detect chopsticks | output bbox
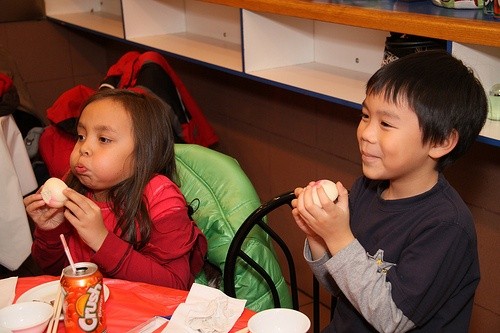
[46,284,62,333]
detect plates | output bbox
[15,280,110,320]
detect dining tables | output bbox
[0,274,258,332]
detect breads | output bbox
[41,177,69,208]
[310,179,338,208]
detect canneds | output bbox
[61,261,107,333]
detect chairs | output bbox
[222,187,350,331]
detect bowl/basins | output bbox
[247,308,311,333]
[0,301,54,333]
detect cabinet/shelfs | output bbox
[43,1,499,148]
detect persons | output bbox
[24,89,207,292]
[291,49,490,333]
